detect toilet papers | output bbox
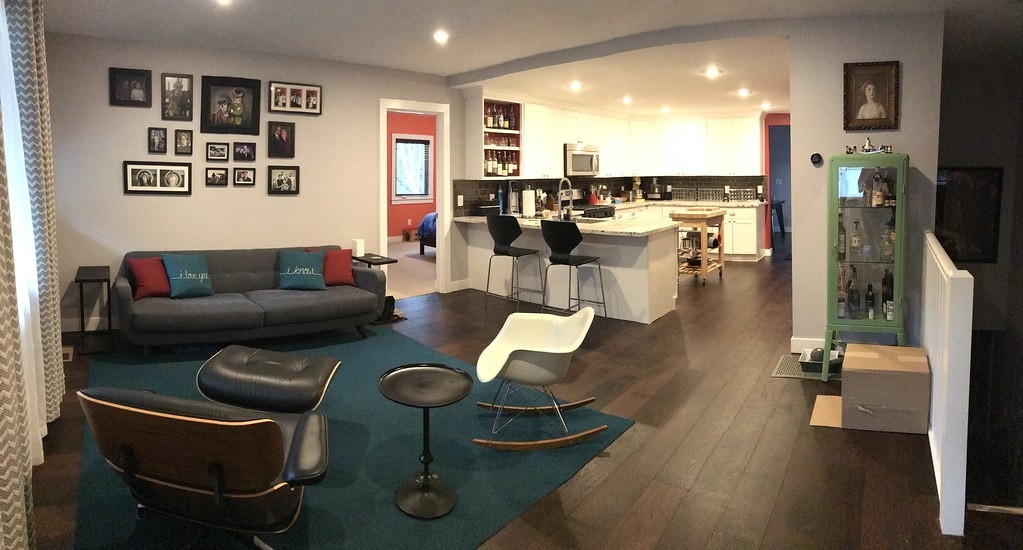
[522,189,536,217]
[352,239,365,257]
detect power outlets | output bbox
[407,219,413,226]
[621,185,625,192]
[489,194,495,201]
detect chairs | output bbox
[473,307,609,453]
[540,219,608,321]
[75,343,343,550]
[485,214,544,313]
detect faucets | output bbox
[557,177,573,221]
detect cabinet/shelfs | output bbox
[612,205,647,220]
[646,206,663,219]
[821,152,908,382]
[670,208,725,287]
[662,207,718,260]
[704,206,758,262]
[464,85,765,187]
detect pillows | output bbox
[324,250,355,286]
[161,252,215,298]
[278,249,327,292]
[126,257,170,300]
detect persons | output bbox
[115,78,146,102]
[273,171,296,192]
[177,133,191,152]
[211,173,216,183]
[237,171,251,182]
[275,91,316,109]
[165,81,189,116]
[151,130,163,151]
[141,172,150,185]
[237,145,252,160]
[271,126,291,156]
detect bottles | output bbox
[484,104,518,177]
[880,224,895,261]
[872,166,885,208]
[838,213,847,260]
[838,264,894,321]
[620,186,625,197]
[849,221,863,258]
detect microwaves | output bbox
[564,143,600,176]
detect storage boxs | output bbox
[401,228,420,242]
[645,192,673,200]
[798,347,840,373]
[808,342,930,435]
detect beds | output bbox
[417,212,437,256]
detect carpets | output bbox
[75,325,636,550]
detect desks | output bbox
[770,199,786,237]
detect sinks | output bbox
[572,217,607,224]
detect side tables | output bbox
[74,266,113,351]
[352,253,405,324]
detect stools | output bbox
[377,360,473,520]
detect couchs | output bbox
[114,244,387,359]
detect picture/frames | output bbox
[843,60,900,132]
[148,127,167,154]
[938,165,1005,264]
[205,167,228,187]
[174,128,193,156]
[233,167,256,186]
[206,141,230,162]
[122,160,194,195]
[268,80,323,116]
[233,141,258,161]
[267,165,300,194]
[200,75,262,136]
[267,120,296,160]
[108,67,153,109]
[161,72,194,122]
